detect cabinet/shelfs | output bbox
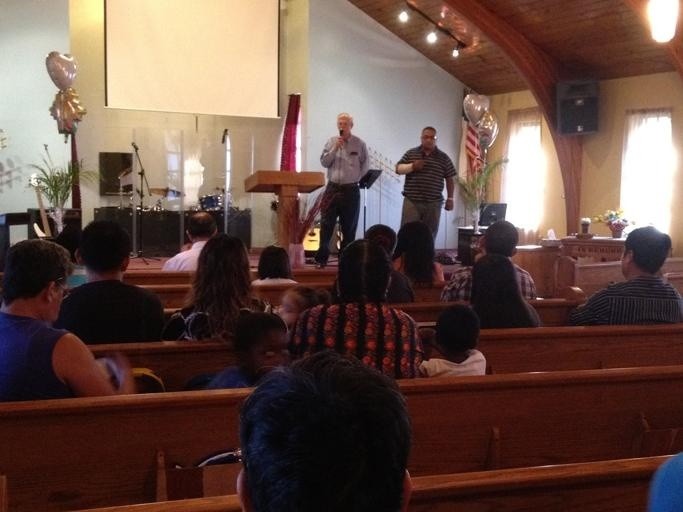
[27,206,83,240]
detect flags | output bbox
[456,111,488,207]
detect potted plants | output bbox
[276,185,340,271]
[450,157,509,234]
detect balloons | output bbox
[46,50,86,136]
[465,91,498,152]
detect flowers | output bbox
[590,207,637,226]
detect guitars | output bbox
[30,173,52,237]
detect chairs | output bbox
[0,268,683,511]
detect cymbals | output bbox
[150,187,185,198]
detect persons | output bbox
[464,253,546,327]
[393,223,446,283]
[164,235,273,344]
[238,351,414,512]
[570,227,683,325]
[395,126,456,235]
[316,115,370,270]
[295,240,423,377]
[442,222,538,301]
[0,239,135,401]
[54,220,167,343]
[367,224,397,256]
[274,285,330,332]
[252,245,301,284]
[421,306,486,377]
[204,313,290,388]
[164,214,218,270]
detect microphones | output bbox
[131,142,139,151]
[339,127,343,136]
[221,129,228,144]
[195,446,243,467]
[420,152,426,160]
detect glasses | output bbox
[420,135,437,140]
[54,280,70,299]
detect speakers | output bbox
[556,79,600,137]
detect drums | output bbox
[199,193,224,211]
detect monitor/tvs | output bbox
[478,203,507,226]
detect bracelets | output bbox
[446,197,454,200]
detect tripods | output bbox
[131,170,162,266]
